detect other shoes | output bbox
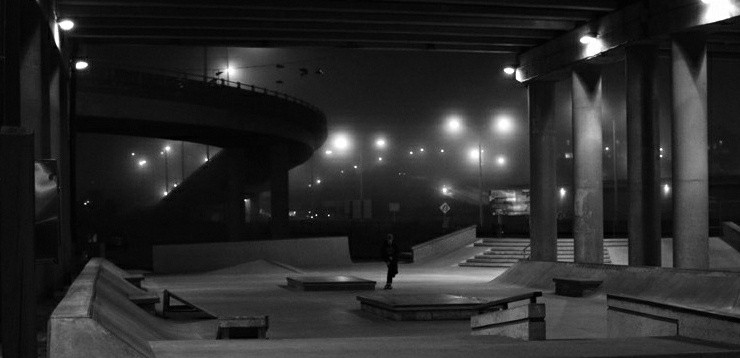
[384,286,391,289]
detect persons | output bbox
[378,234,399,289]
